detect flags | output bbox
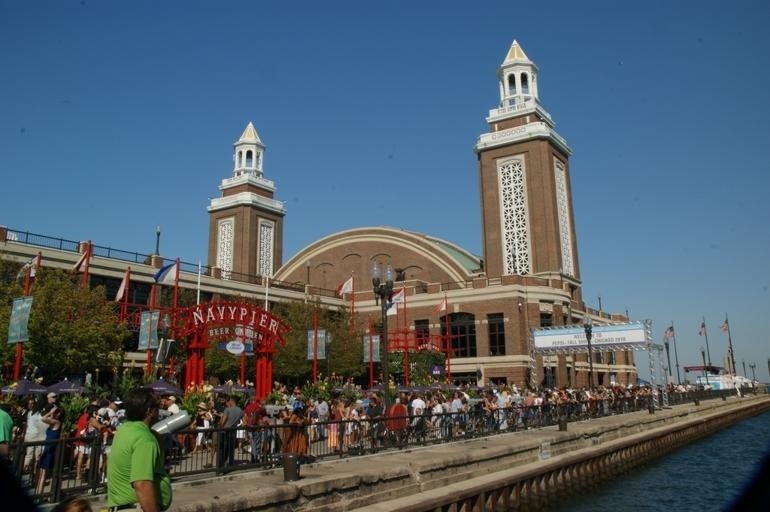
[334,275,446,319]
[665,327,674,337]
[14,251,177,304]
[721,320,728,332]
[698,322,706,336]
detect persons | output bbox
[657,382,697,394]
[0,388,217,511]
[547,383,652,427]
[208,379,409,475]
[411,381,554,444]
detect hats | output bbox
[197,401,207,409]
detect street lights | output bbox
[583,315,594,391]
[748,361,756,382]
[307,259,310,285]
[156,226,161,254]
[700,347,708,384]
[664,336,672,376]
[372,263,394,403]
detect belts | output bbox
[107,503,138,512]
